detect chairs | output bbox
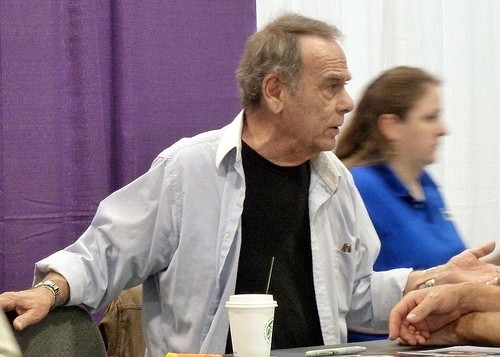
[5,284,145,357]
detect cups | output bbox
[225,294,278,357]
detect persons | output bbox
[335,64,475,343]
[0,11,500,356]
[388,281,500,349]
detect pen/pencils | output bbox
[305,346,366,357]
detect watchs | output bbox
[30,280,61,312]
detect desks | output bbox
[224,340,500,357]
